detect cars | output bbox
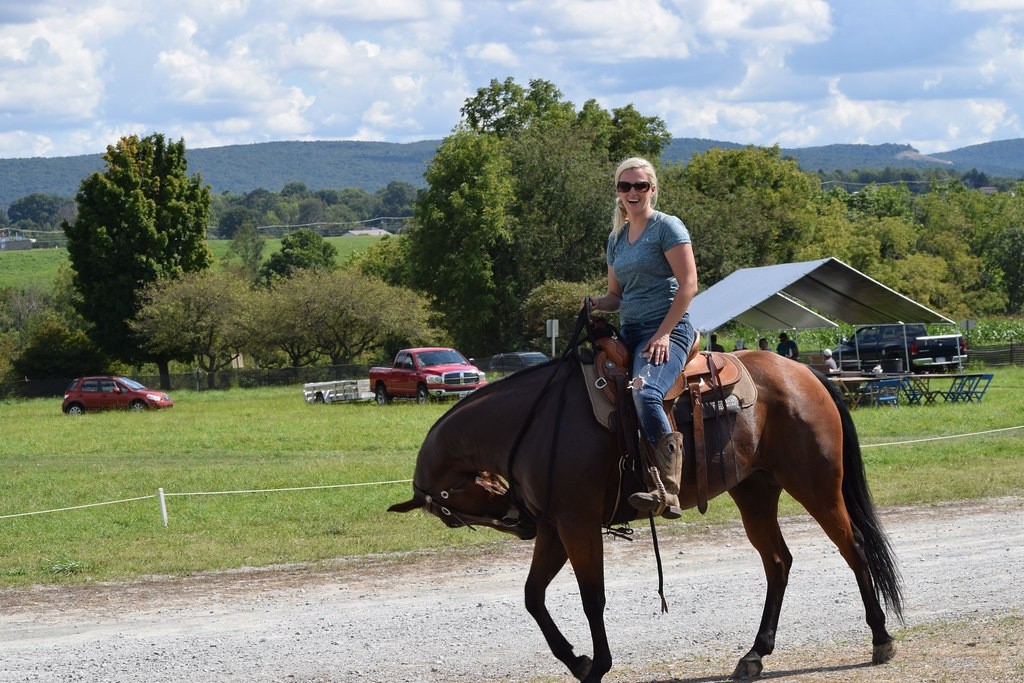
[62,375,174,416]
[488,350,552,375]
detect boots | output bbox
[628,432,683,519]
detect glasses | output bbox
[617,181,652,193]
[780,339,785,342]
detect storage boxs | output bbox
[810,364,830,373]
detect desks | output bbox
[924,361,959,373]
[827,376,888,408]
[902,373,973,403]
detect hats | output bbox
[736,342,743,349]
[824,349,833,356]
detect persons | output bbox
[822,348,837,371]
[777,331,800,361]
[580,155,698,520]
[758,337,770,352]
[703,334,725,354]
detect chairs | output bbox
[834,378,899,407]
[901,374,993,405]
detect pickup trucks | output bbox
[830,321,968,374]
[369,347,489,405]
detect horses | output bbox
[386,317,907,682]
